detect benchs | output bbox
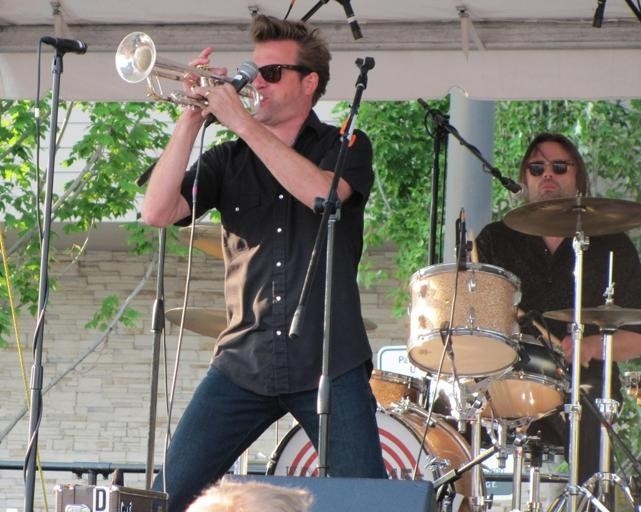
[1,462,568,486]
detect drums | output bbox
[369,371,426,411]
[462,334,568,428]
[408,263,523,379]
[266,399,488,511]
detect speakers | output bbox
[221,473,440,512]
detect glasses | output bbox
[524,160,575,176]
[257,65,311,83]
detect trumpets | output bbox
[115,32,266,117]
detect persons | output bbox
[185,479,314,512]
[140,14,389,512]
[468,133,641,512]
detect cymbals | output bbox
[163,308,228,339]
[502,197,641,237]
[179,224,223,260]
[542,303,640,328]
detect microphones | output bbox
[204,60,261,128]
[500,176,529,201]
[40,34,89,56]
[339,0,364,40]
[454,218,470,273]
